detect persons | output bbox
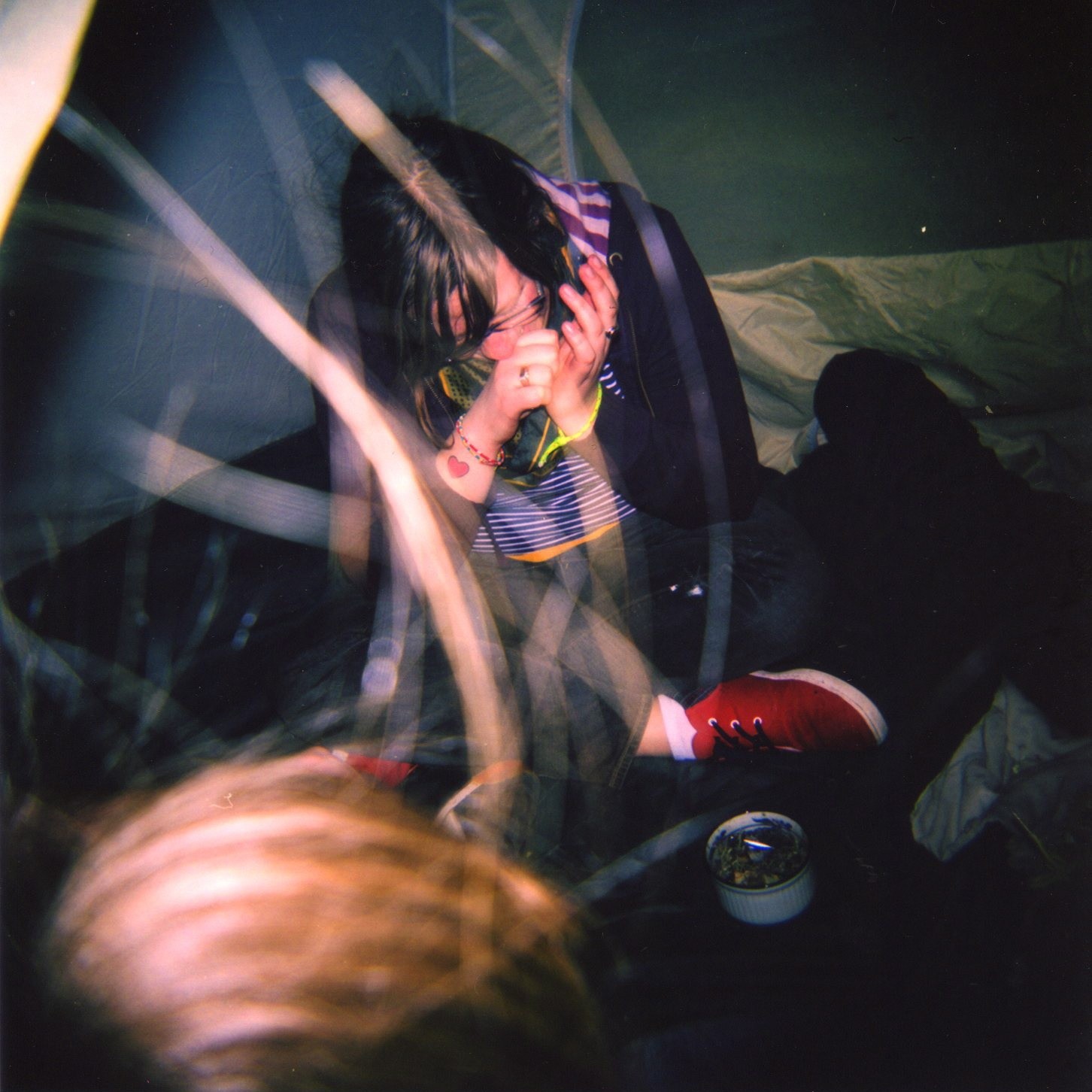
[306,117,888,798]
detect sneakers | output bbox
[685,668,889,763]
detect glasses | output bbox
[446,277,547,361]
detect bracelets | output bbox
[454,412,507,469]
[536,379,602,469]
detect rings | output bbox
[518,363,531,387]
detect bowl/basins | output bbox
[705,810,815,927]
